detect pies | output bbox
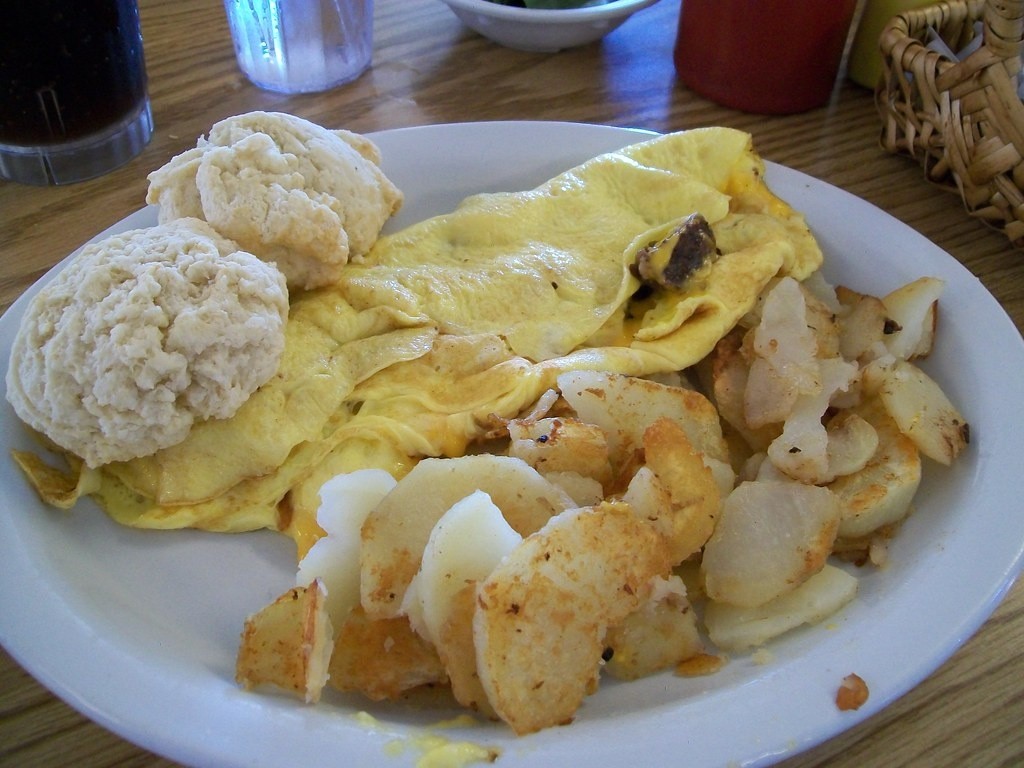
[10,124,827,533]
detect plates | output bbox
[0,121,1024,768]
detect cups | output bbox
[224,0,374,95]
[0,0,154,186]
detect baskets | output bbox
[876,0,1024,250]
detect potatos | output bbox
[232,277,973,737]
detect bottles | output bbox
[845,0,937,96]
[674,0,855,113]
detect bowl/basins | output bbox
[442,1,665,49]
